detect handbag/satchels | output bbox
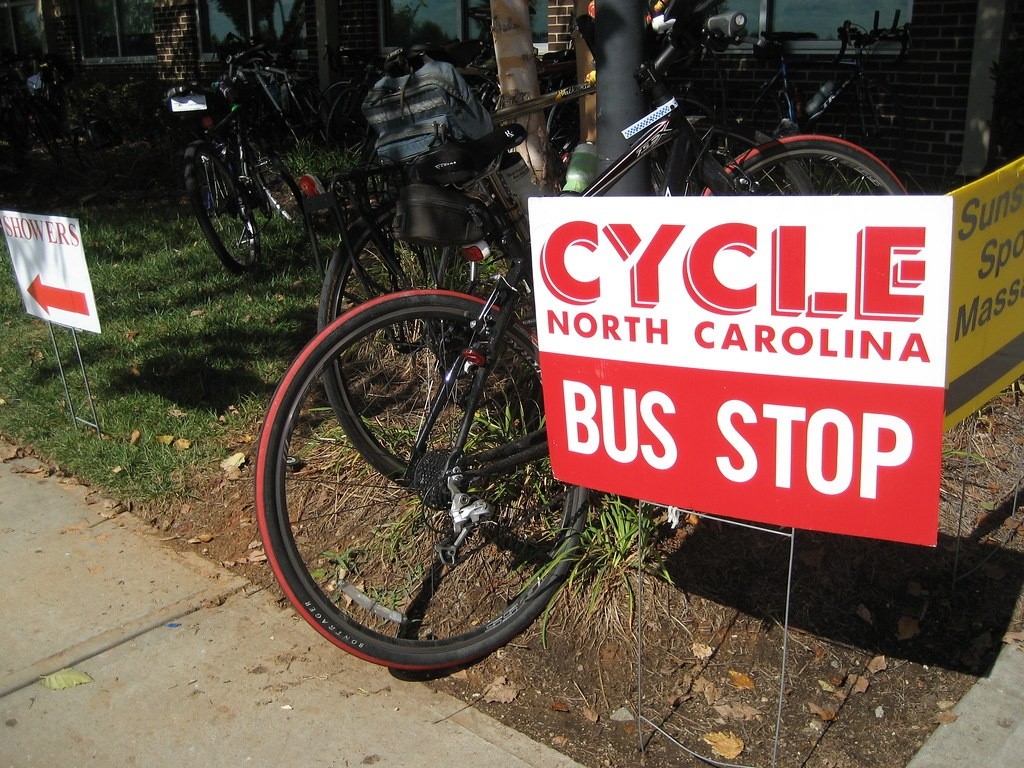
[410,140,475,184]
[359,51,493,164]
[389,184,493,244]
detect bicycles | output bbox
[164,34,307,278]
[0,52,119,178]
[252,1,927,675]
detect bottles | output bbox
[558,138,598,196]
[499,151,545,216]
